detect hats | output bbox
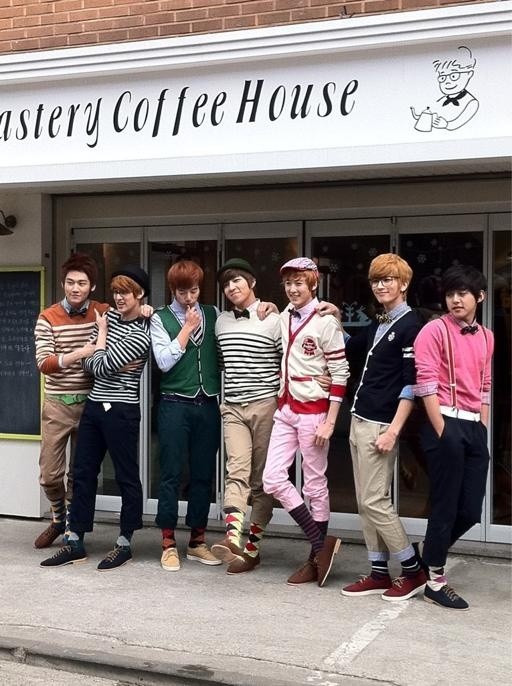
[279,257,318,272]
[112,264,150,297]
[216,259,257,279]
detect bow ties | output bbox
[461,326,478,335]
[376,314,392,324]
[288,309,301,319]
[232,309,249,319]
[68,308,87,319]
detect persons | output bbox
[314,252,433,603]
[38,265,153,571]
[208,257,334,575]
[412,263,496,612]
[257,255,351,587]
[32,254,155,550]
[141,258,224,572]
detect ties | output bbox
[193,325,203,345]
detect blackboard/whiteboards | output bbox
[0,269,45,442]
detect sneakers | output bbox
[36,522,66,548]
[97,543,132,571]
[422,584,470,611]
[186,543,222,565]
[341,573,393,596]
[161,545,180,572]
[314,535,342,587]
[62,528,70,548]
[411,542,423,567]
[382,569,427,602]
[287,560,318,585]
[39,545,87,568]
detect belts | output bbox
[439,405,481,422]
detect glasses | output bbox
[367,276,400,288]
